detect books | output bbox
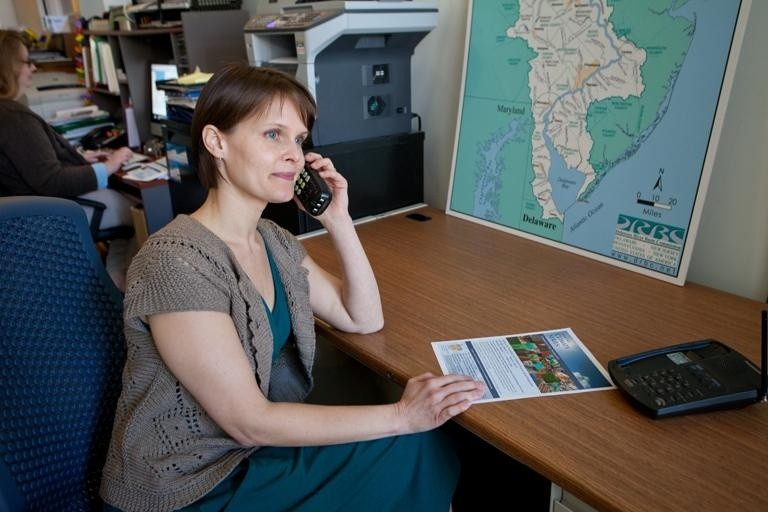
[71,33,120,93]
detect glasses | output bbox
[21,58,31,67]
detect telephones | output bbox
[80,124,128,151]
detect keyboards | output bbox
[104,147,151,166]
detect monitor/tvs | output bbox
[148,63,178,121]
[260,58,299,78]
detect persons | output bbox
[0,28,140,294]
[93,63,487,512]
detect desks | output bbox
[70,10,249,237]
[293,205,768,512]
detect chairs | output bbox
[0,194,125,512]
[0,150,135,269]
